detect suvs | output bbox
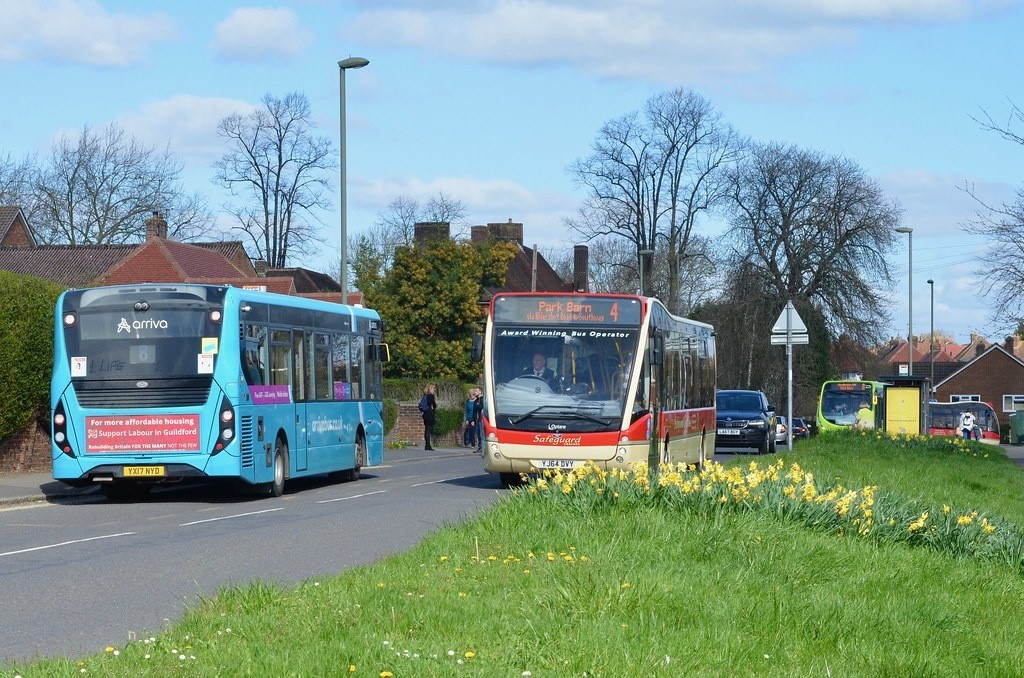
[715,388,777,455]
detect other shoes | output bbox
[473,448,483,453]
[425,446,436,451]
[466,444,471,448]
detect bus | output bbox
[927,401,1001,447]
[815,378,893,439]
[471,291,717,488]
[49,282,390,500]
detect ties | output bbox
[537,372,540,377]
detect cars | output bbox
[775,415,810,444]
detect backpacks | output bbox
[964,414,971,426]
[419,395,430,411]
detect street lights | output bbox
[927,279,934,399]
[337,56,371,306]
[894,226,913,376]
[639,249,655,296]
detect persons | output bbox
[521,351,554,388]
[462,387,483,453]
[834,403,844,413]
[973,420,982,441]
[938,420,943,427]
[959,408,975,439]
[855,401,874,429]
[584,354,602,390]
[252,356,261,375]
[422,382,437,451]
